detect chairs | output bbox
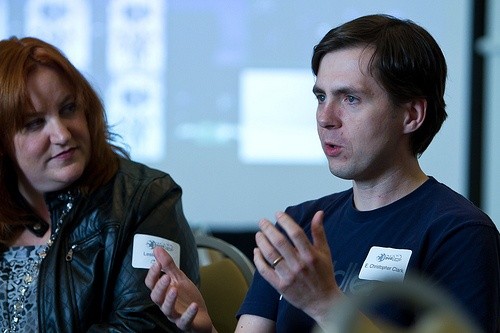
[195,233,256,333]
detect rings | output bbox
[270,254,283,269]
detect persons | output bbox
[139,12,500,333]
[0,36,206,332]
